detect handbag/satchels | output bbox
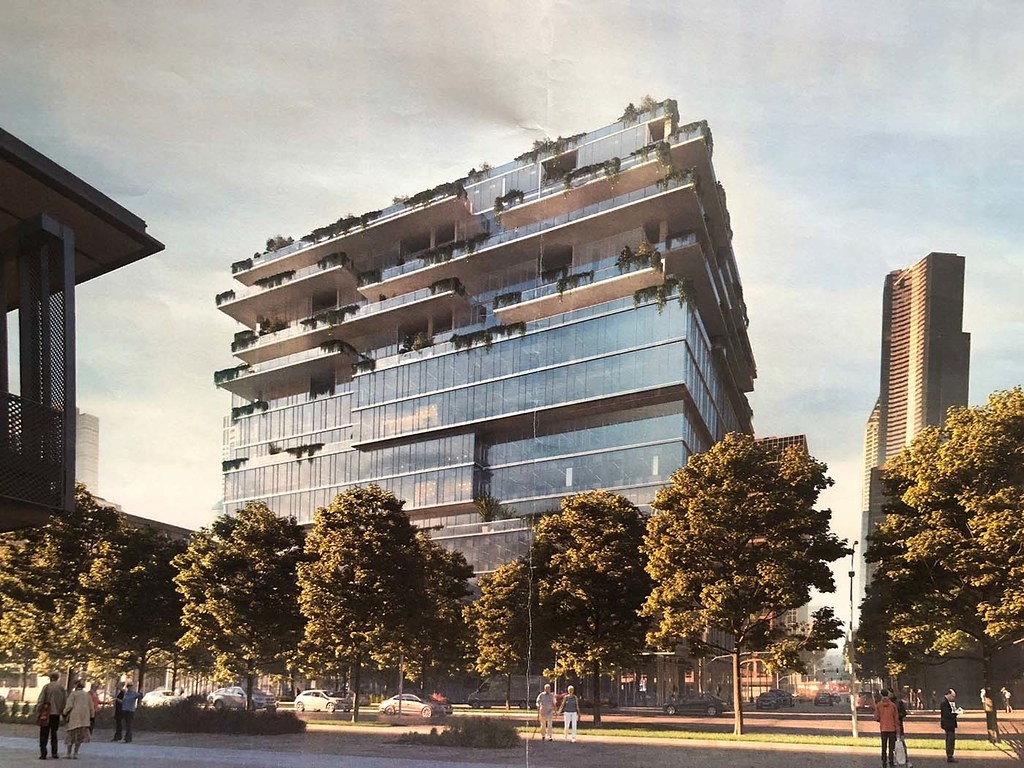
[37,683,50,727]
[894,736,906,766]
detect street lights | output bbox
[848,539,858,708]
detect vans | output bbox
[468,673,551,711]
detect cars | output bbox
[756,687,842,707]
[857,691,874,710]
[89,689,454,720]
[665,691,730,716]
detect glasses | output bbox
[127,685,133,688]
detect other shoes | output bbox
[63,754,71,759]
[542,737,545,740]
[564,739,568,741]
[123,738,132,743]
[549,738,552,741]
[571,740,576,743]
[112,739,118,742]
[39,756,46,760]
[73,753,80,759]
[52,754,59,759]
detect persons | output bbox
[939,688,964,764]
[37,672,66,760]
[886,687,914,768]
[122,682,144,743]
[536,683,558,742]
[558,685,582,743]
[87,682,109,741]
[110,681,126,743]
[62,679,95,759]
[984,687,1001,743]
[905,685,1015,715]
[873,689,900,768]
